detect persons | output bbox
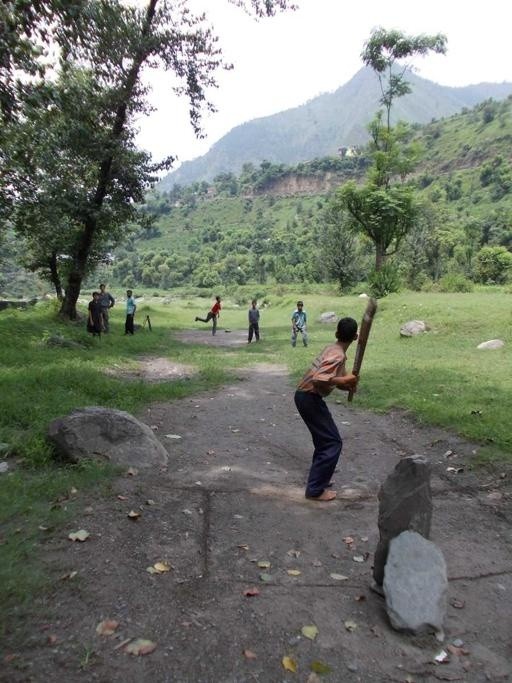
[195,294,222,335]
[97,283,116,334]
[290,300,309,348]
[292,316,362,500]
[85,291,106,341]
[246,298,260,343]
[123,288,137,335]
